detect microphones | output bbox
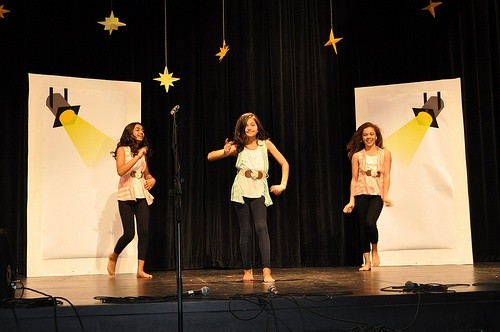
[170,105,180,114]
[184,285,210,296]
[405,280,430,288]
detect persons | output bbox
[208,113,289,282]
[107,122,156,278]
[343,122,394,271]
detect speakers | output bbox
[0,262,17,301]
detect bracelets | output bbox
[135,155,141,159]
